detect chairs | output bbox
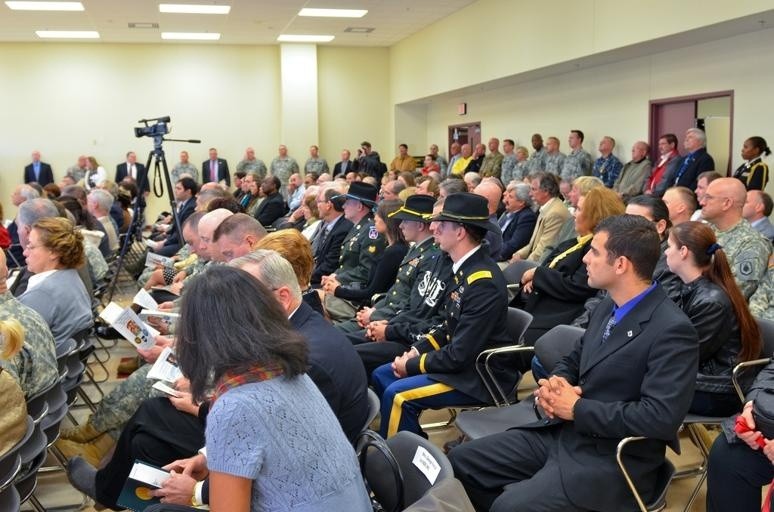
[454,305,590,446]
[1,219,143,511]
[665,314,771,507]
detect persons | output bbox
[318,129,773,512]
[0,146,375,511]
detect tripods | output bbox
[106,150,185,303]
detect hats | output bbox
[331,181,501,235]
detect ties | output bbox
[600,317,616,343]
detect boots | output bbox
[52,326,140,503]
